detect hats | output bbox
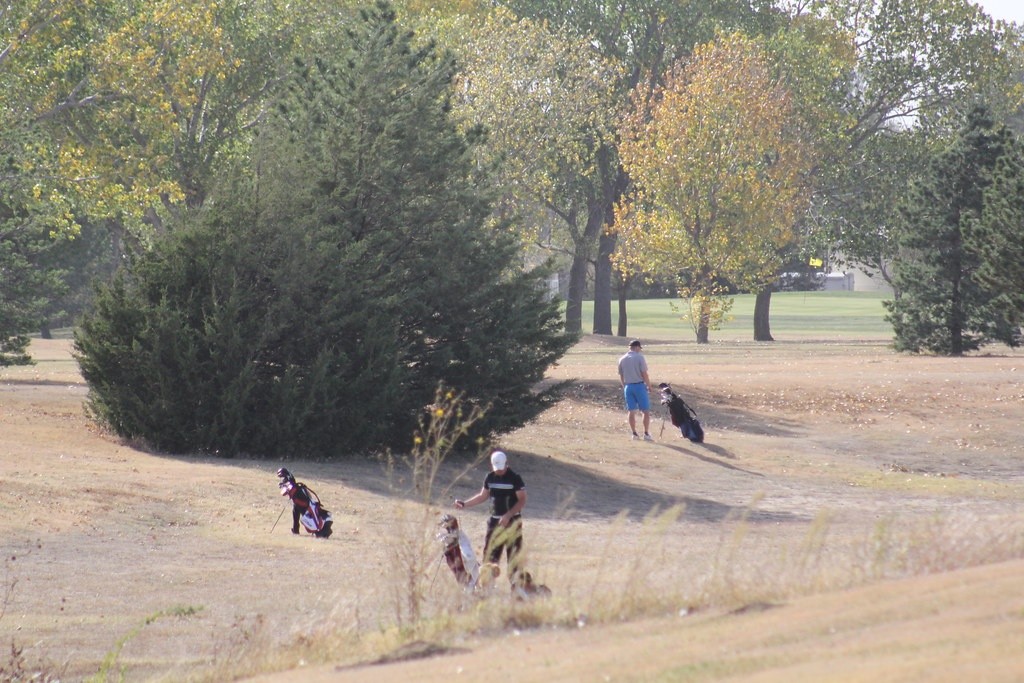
[629,341,643,350]
[491,452,506,471]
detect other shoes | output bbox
[632,434,640,441]
[644,434,652,441]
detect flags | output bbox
[809,257,822,267]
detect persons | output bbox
[452,451,527,585]
[617,340,653,440]
